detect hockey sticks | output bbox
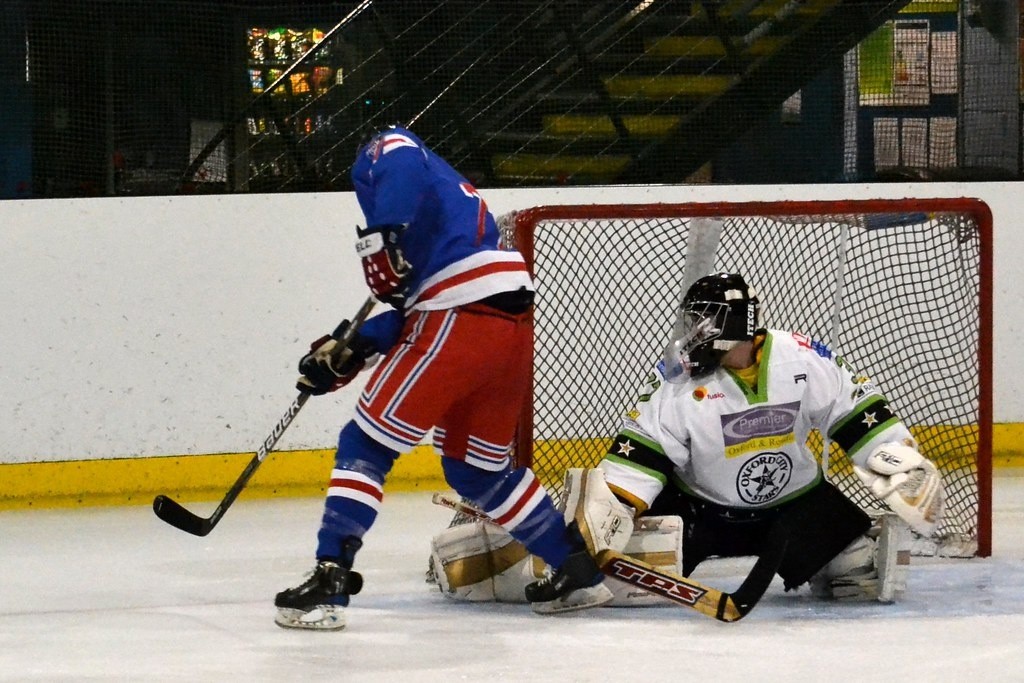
[151,293,385,538]
[431,490,793,624]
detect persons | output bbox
[557,271,945,604]
[274,122,617,634]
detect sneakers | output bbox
[524,518,615,614]
[273,532,362,631]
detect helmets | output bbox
[356,124,398,153]
[663,271,764,380]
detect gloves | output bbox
[354,224,418,309]
[295,320,381,396]
